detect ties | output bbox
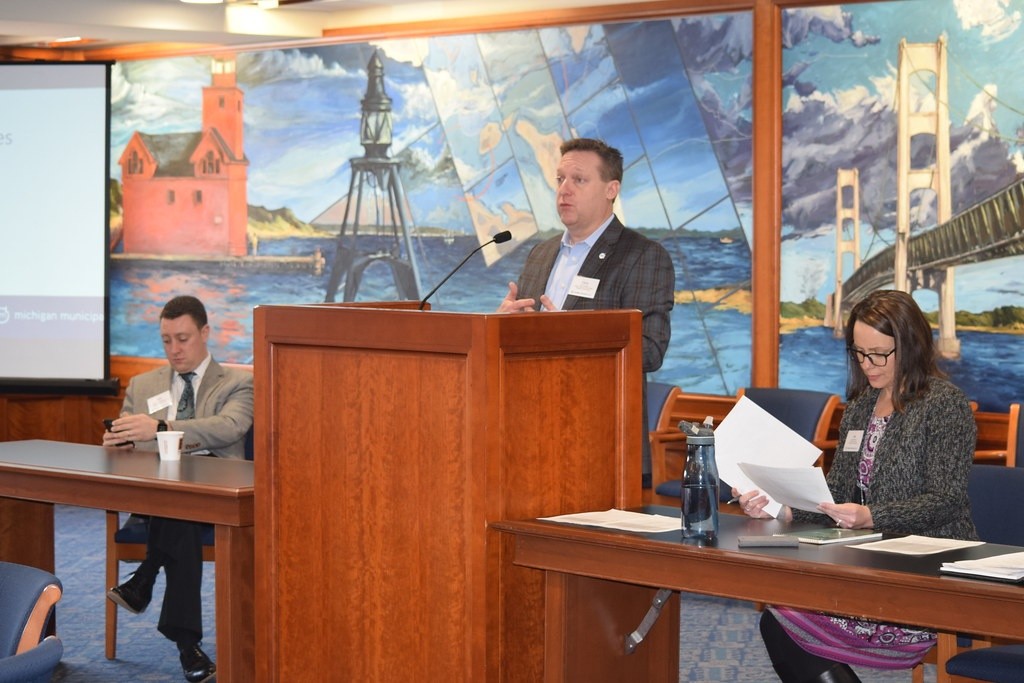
[176,369,196,421]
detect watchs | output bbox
[157,420,167,431]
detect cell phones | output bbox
[103,418,135,448]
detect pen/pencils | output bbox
[727,495,742,504]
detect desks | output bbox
[492,503,1024,683]
[0,440,256,683]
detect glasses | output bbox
[846,343,898,366]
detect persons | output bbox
[102,295,253,683]
[497,139,675,486]
[731,290,976,683]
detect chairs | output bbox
[0,561,64,683]
[106,427,254,659]
[643,381,1024,682]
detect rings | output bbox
[128,432,130,436]
[839,520,842,524]
[836,523,839,526]
[744,507,749,511]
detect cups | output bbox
[156,431,185,461]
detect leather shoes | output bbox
[106,569,149,616]
[178,642,216,683]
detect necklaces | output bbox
[860,392,879,506]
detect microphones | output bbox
[418,231,512,310]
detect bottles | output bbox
[678,416,719,542]
